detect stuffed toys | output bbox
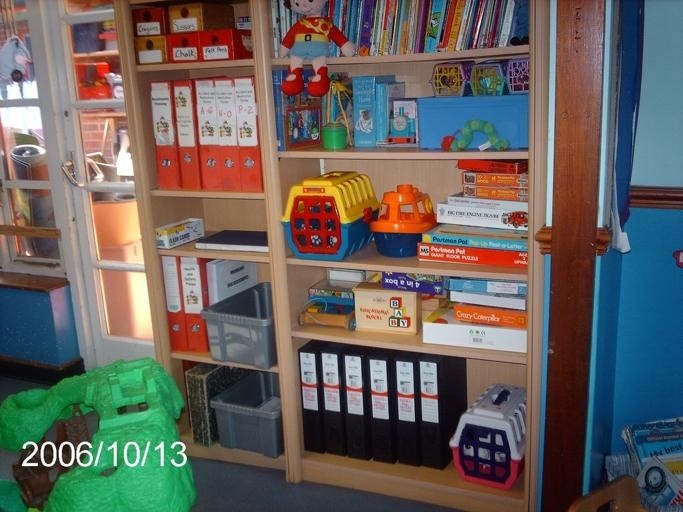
[281,1,354,98]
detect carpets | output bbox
[188,456,461,512]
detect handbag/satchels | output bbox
[13,401,100,511]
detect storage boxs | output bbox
[210,373,283,459]
[198,282,277,369]
[130,4,250,64]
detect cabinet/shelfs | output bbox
[117,0,285,469]
[257,2,551,512]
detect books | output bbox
[423,278,527,355]
[417,159,528,269]
[195,229,268,251]
[270,0,528,56]
